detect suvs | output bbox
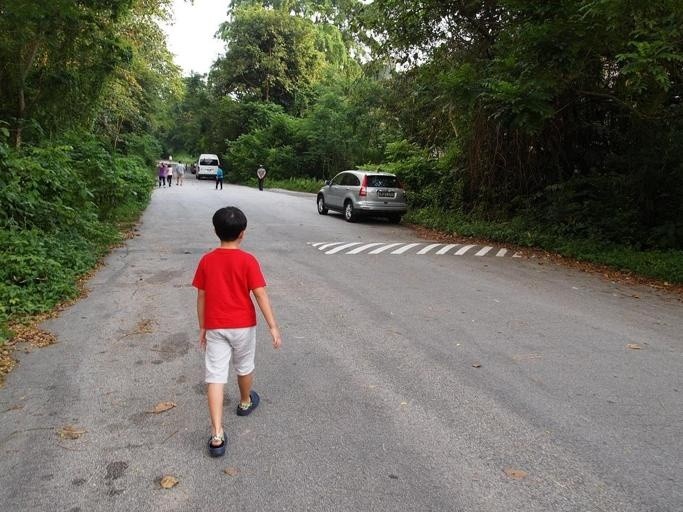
[314,168,410,226]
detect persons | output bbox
[159,162,165,187]
[166,164,172,187]
[175,161,185,185]
[256,164,266,191]
[215,165,223,190]
[191,206,282,456]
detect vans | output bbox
[195,152,221,181]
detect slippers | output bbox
[236,390,260,416]
[207,431,228,456]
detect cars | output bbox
[190,161,196,175]
